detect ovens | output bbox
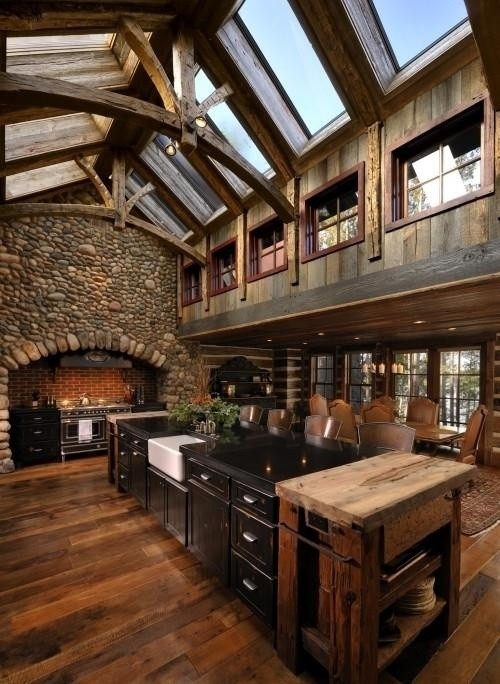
[61,415,108,458]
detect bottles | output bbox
[31,389,39,409]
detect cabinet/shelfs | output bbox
[277,449,477,683]
[231,476,277,632]
[186,457,231,590]
[129,433,146,509]
[117,427,129,494]
[215,369,271,386]
[13,409,61,463]
[147,466,187,546]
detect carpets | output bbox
[460,466,500,535]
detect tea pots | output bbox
[78,393,91,407]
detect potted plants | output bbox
[30,389,40,406]
[168,394,240,432]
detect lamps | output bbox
[164,138,178,156]
[186,112,207,134]
[362,341,405,379]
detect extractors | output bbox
[59,350,133,369]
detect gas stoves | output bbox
[60,405,111,418]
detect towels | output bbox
[78,419,93,443]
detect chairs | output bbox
[239,392,489,488]
